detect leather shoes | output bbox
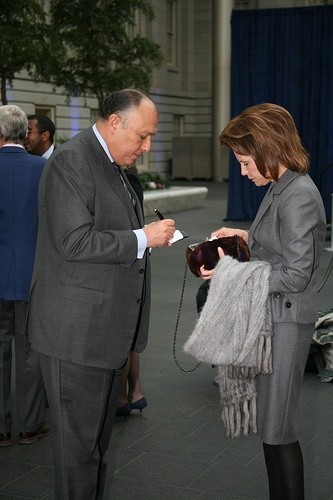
[19,422,49,444]
[0,432,13,446]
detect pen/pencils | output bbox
[155,209,164,220]
[149,247,151,255]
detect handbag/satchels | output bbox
[186,236,250,278]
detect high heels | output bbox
[128,399,146,413]
[113,404,130,420]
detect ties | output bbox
[112,161,136,207]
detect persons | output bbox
[114,160,154,421]
[200,101,328,500]
[25,114,58,161]
[0,104,52,448]
[23,87,178,500]
